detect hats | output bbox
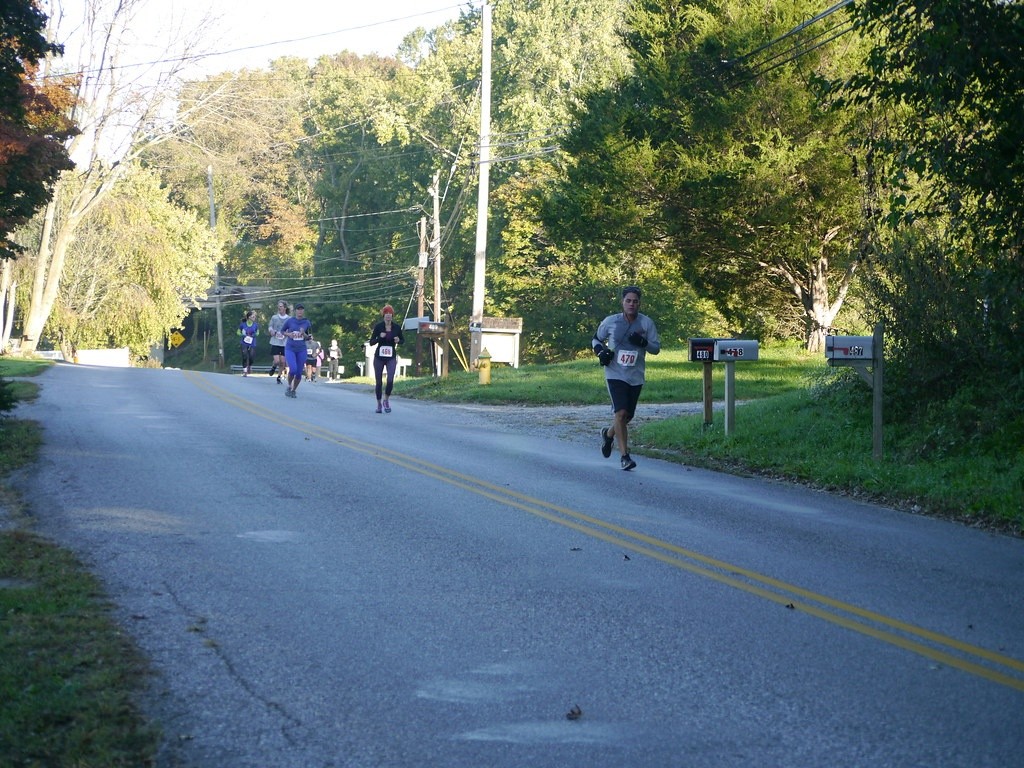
[307,334,313,341]
[622,286,641,298]
[295,304,305,309]
[383,305,394,317]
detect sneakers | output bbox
[376,404,382,413]
[621,453,636,470]
[383,400,391,412]
[601,427,614,458]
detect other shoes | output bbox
[242,372,247,376]
[286,387,291,396]
[292,391,296,398]
[277,378,281,384]
[269,370,275,375]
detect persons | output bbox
[370,305,405,413]
[316,342,324,377]
[268,300,291,384]
[281,304,313,398]
[306,334,322,382]
[592,287,659,470]
[327,339,343,382]
[237,311,260,377]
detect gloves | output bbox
[599,350,611,366]
[629,332,646,347]
